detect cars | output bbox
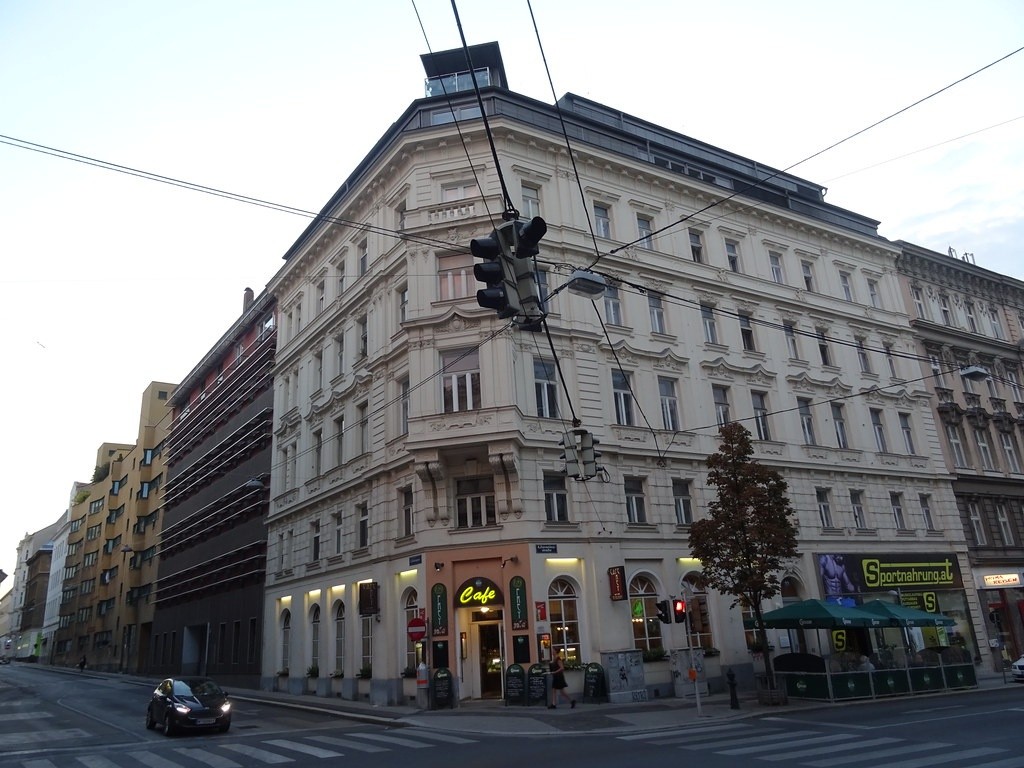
[147,675,233,735]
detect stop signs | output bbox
[406,618,426,642]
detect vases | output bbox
[330,678,343,694]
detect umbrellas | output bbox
[850,598,957,655]
[744,599,890,657]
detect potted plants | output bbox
[435,563,444,571]
[751,640,766,673]
[276,667,289,691]
[400,665,417,697]
[355,662,372,694]
[306,663,319,691]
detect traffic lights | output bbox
[674,599,686,623]
[469,230,518,320]
[512,214,549,258]
[655,600,671,625]
[557,427,603,484]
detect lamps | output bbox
[960,366,989,385]
[122,544,132,554]
[501,557,517,568]
[567,270,607,300]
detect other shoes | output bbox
[548,704,556,710]
[569,700,577,709]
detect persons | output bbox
[818,554,854,600]
[547,646,576,710]
[830,653,929,695]
[80,655,86,672]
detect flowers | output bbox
[329,670,344,679]
